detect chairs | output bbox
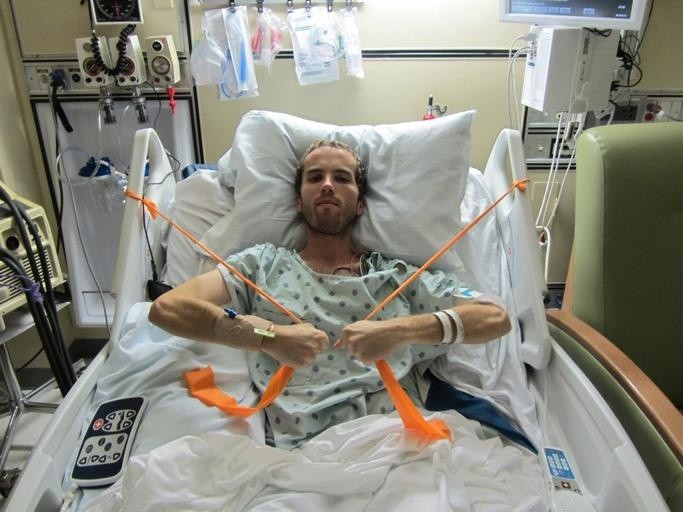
[542,120,683,512]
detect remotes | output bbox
[538,447,596,512]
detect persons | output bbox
[148,136,512,451]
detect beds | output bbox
[1,107,674,511]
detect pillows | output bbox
[194,110,476,263]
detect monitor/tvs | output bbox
[498,0,651,31]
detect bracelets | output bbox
[432,308,465,346]
